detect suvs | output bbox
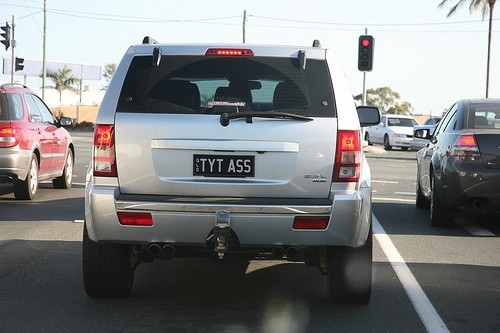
[83,36,381,304]
[0,82,75,200]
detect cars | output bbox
[410,98,500,228]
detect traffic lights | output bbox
[15,57,24,71]
[358,35,375,70]
[0,22,10,50]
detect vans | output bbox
[362,114,422,151]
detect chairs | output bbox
[214,85,252,108]
[271,83,309,108]
[150,81,200,111]
[475,116,488,125]
